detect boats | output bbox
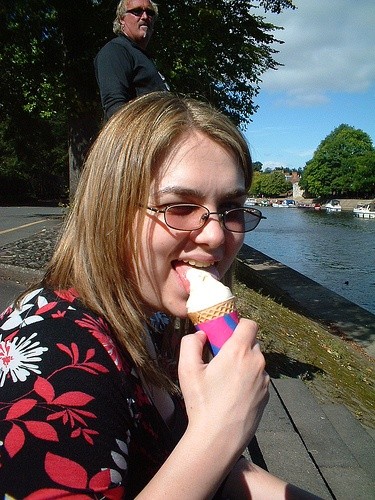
[245,198,375,219]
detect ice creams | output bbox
[184,269,239,358]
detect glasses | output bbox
[137,202,267,233]
[124,6,157,17]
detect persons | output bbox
[95,0,172,120]
[0,91,331,500]
